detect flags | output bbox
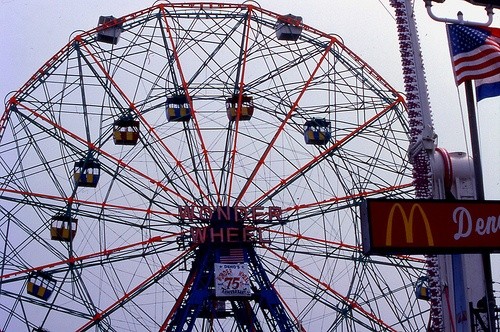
[474,74,500,103]
[446,24,500,87]
